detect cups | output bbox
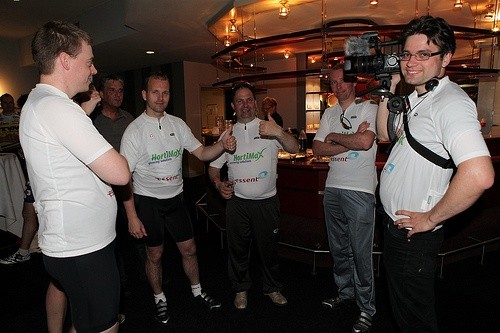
[216,116,233,133]
[307,124,319,131]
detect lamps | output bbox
[455,0,463,8]
[279,0,290,16]
[283,50,290,59]
[224,35,231,47]
[484,4,493,20]
[230,19,237,33]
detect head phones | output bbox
[424,74,446,92]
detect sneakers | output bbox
[234,288,248,309]
[264,291,288,306]
[155,297,170,323]
[322,297,355,311]
[353,312,372,333]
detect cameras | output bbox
[343,32,400,89]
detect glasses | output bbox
[400,51,442,61]
[340,111,352,130]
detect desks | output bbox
[1,152,28,237]
[276,159,329,237]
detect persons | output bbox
[376,15,496,333]
[312,64,378,333]
[92,74,154,298]
[0,21,131,333]
[263,96,284,128]
[120,73,236,324]
[209,82,300,308]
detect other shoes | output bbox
[0,251,31,264]
[194,289,221,309]
[119,314,125,325]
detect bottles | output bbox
[297,128,307,154]
